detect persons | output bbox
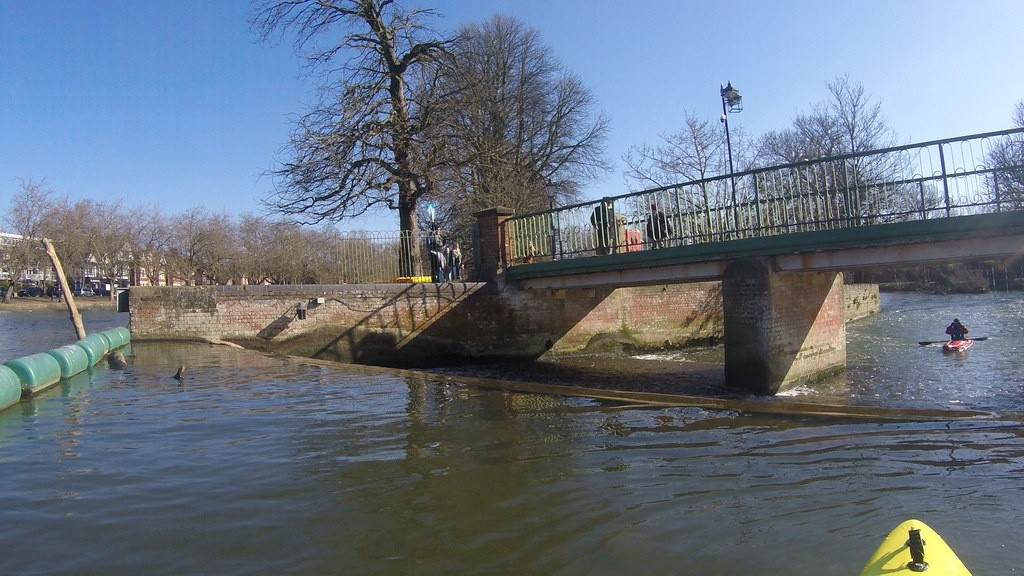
[427,220,465,283]
[644,205,674,249]
[590,196,615,254]
[945,318,969,341]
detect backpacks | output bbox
[951,324,963,340]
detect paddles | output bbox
[918,337,987,345]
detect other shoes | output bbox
[433,280,445,283]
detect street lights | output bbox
[721,80,744,237]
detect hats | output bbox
[649,205,656,209]
[432,224,439,231]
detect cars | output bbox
[74,288,93,298]
[46,286,60,298]
[0,286,14,298]
[18,287,43,297]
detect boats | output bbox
[943,339,974,352]
